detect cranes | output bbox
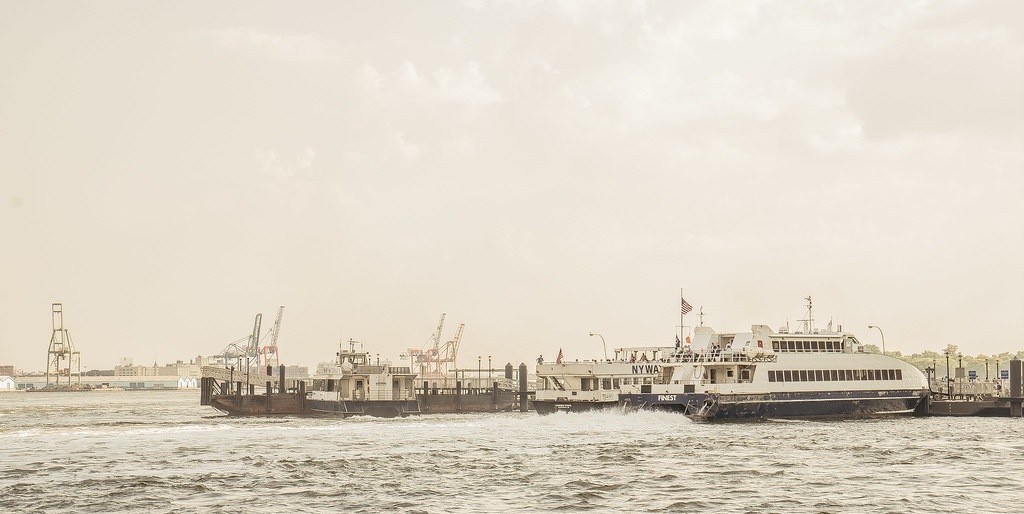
[214,305,285,373]
[414,313,466,378]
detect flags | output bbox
[676,335,680,347]
[682,298,693,315]
[557,347,563,364]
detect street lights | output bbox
[589,332,607,360]
[985,358,989,380]
[868,326,885,355]
[933,359,936,378]
[478,356,481,394]
[996,359,999,379]
[489,356,491,389]
[958,355,964,400]
[944,352,951,400]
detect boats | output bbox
[616,295,931,424]
[305,338,421,418]
[531,288,717,417]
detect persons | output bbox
[349,359,354,363]
[640,353,648,362]
[538,355,543,365]
[630,354,636,363]
[674,342,731,362]
